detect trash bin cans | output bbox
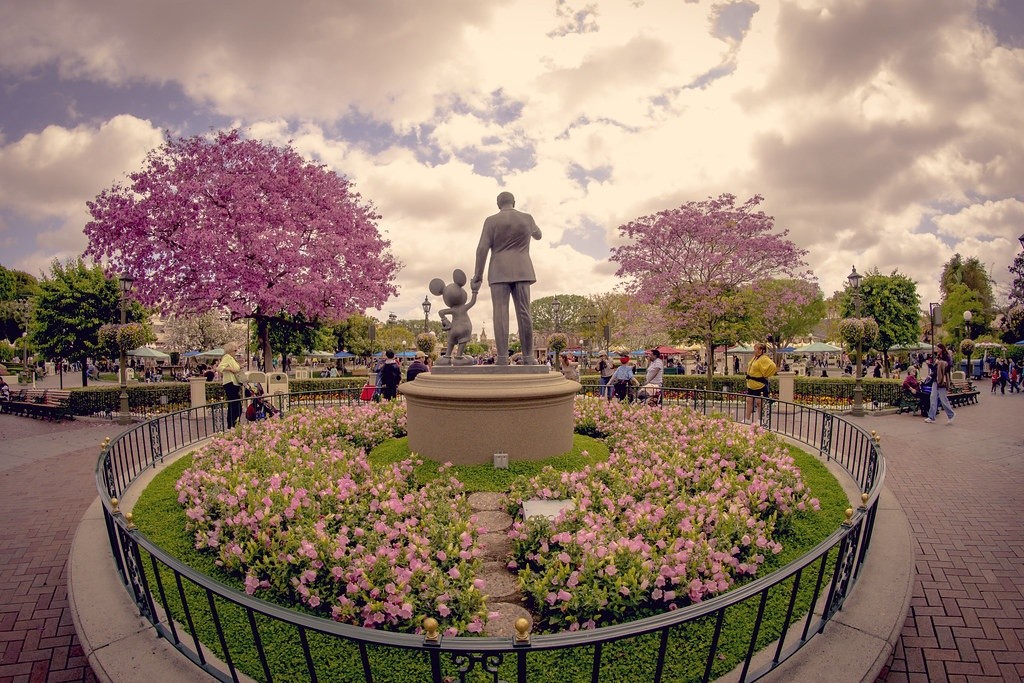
[45,362,55,376]
[791,363,806,377]
[118,368,135,383]
[242,371,267,412]
[959,358,983,380]
[266,372,289,411]
[295,367,310,378]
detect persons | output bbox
[887,343,956,423]
[126,358,222,382]
[374,350,428,403]
[862,354,883,379]
[546,355,589,380]
[473,192,542,366]
[312,357,372,378]
[981,352,1024,394]
[55,359,120,378]
[594,351,664,406]
[781,354,855,377]
[735,356,739,372]
[742,344,776,424]
[0,377,10,412]
[478,355,522,365]
[667,353,718,375]
[257,357,292,372]
[217,343,247,430]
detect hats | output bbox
[907,366,914,372]
[598,350,607,357]
[620,355,629,363]
[415,351,428,359]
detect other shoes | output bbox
[755,419,763,427]
[741,419,751,424]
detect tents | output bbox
[196,348,226,366]
[126,348,170,365]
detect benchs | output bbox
[350,369,368,376]
[2,390,74,423]
[897,380,980,416]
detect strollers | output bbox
[637,382,663,407]
[240,380,285,423]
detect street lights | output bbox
[422,295,431,332]
[116,265,135,425]
[963,311,973,386]
[23,332,27,368]
[848,265,864,416]
[579,340,583,368]
[808,333,812,344]
[552,298,560,332]
[402,340,406,364]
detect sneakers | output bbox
[948,413,956,423]
[924,417,936,423]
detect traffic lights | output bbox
[776,336,780,342]
[603,326,609,340]
[767,336,772,342]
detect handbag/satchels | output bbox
[246,404,256,421]
[234,370,248,384]
